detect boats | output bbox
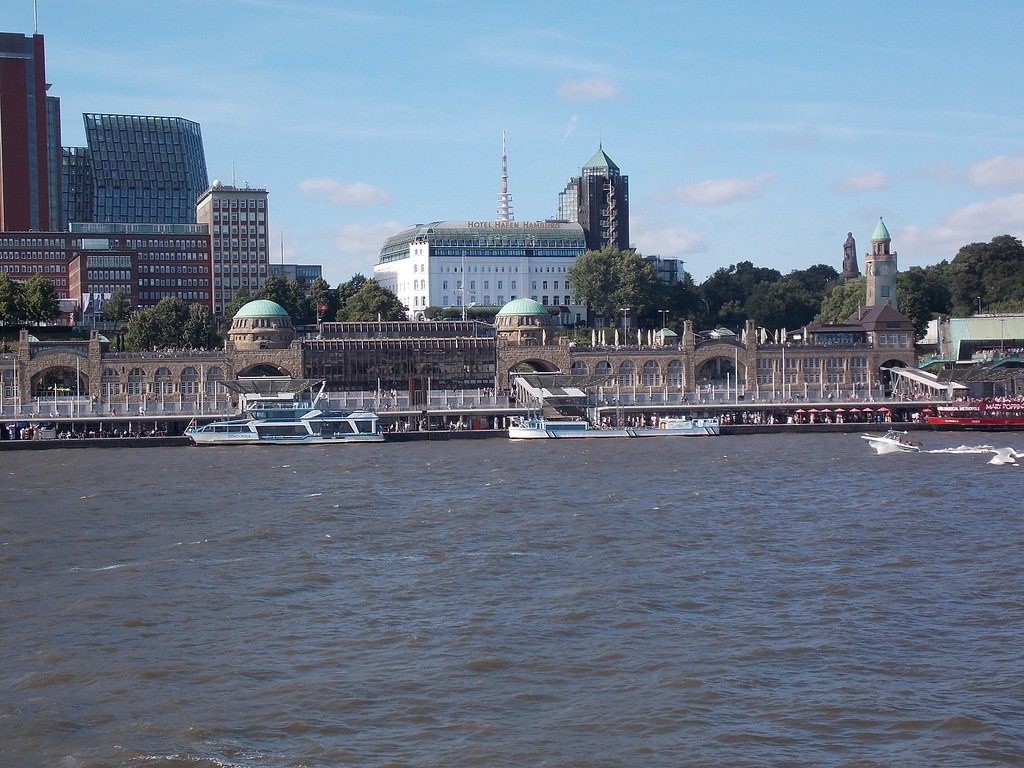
[507,410,720,438]
[861,427,921,454]
[184,409,385,445]
[926,401,1024,431]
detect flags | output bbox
[316,299,325,312]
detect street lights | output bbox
[659,309,669,328]
[621,308,631,346]
[999,319,1006,353]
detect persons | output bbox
[381,420,477,430]
[905,441,913,446]
[795,408,805,424]
[602,411,793,429]
[856,393,859,402]
[796,393,799,401]
[910,409,954,424]
[846,395,856,410]
[841,233,862,272]
[958,394,1024,416]
[976,347,1024,358]
[794,414,891,423]
[484,387,506,396]
[5,409,167,438]
[827,392,834,402]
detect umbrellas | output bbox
[835,408,846,412]
[821,408,832,416]
[849,408,860,419]
[808,408,819,414]
[862,408,874,423]
[877,407,891,422]
